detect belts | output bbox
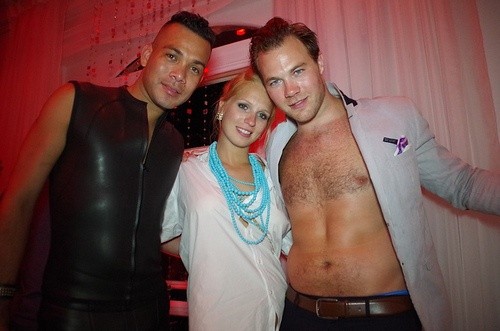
[286,283,413,320]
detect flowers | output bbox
[394,135,409,155]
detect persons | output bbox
[157,65,294,331]
[0,10,215,331]
[247,15,500,331]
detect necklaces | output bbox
[208,140,272,245]
